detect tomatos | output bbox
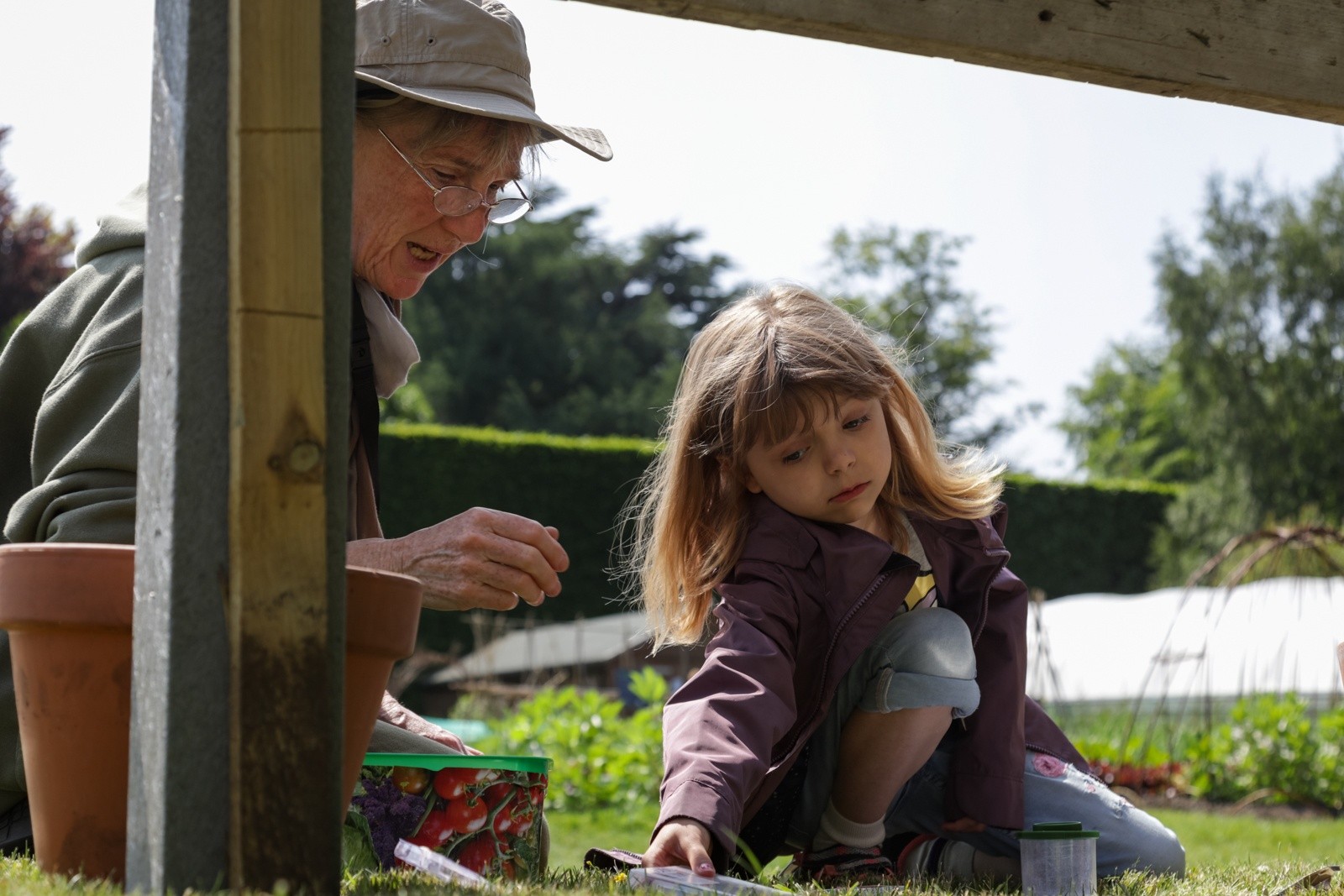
[392,767,549,875]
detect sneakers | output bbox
[801,845,906,896]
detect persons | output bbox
[635,282,1186,882]
[0,0,615,859]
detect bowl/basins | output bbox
[340,752,556,882]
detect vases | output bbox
[0,544,424,895]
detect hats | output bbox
[355,0,614,162]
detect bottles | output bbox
[1010,819,1101,895]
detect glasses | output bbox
[357,108,534,225]
